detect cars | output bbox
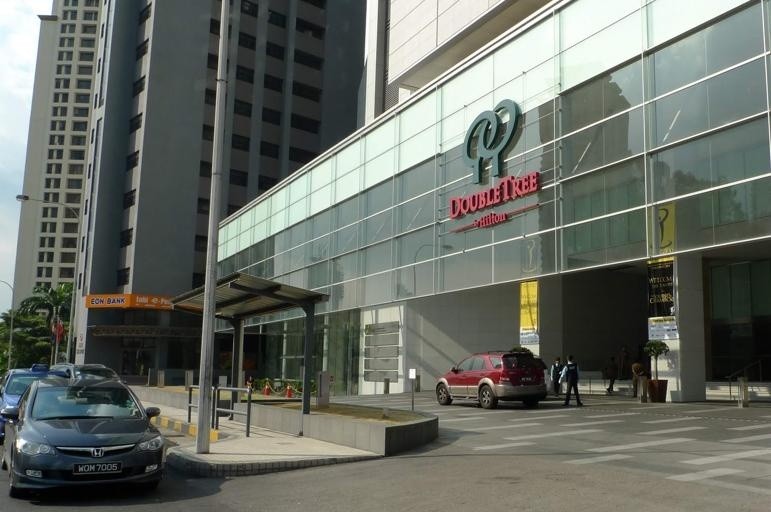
[0,364,167,496]
[436,350,547,409]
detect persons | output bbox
[550,356,564,397]
[631,356,645,398]
[558,354,584,406]
[602,355,619,394]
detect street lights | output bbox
[414,244,452,296]
[16,195,80,363]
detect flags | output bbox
[49,316,56,346]
[55,312,65,345]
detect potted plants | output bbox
[644,340,669,403]
